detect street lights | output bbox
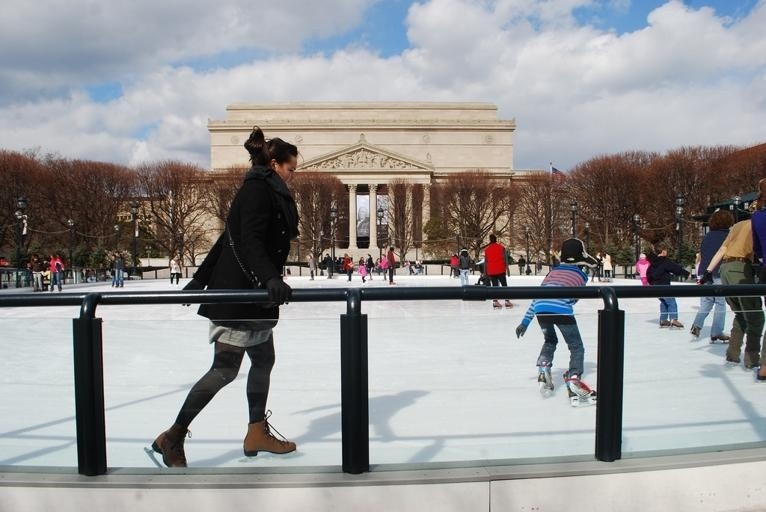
[583,220,590,276]
[66,217,75,269]
[674,193,684,264]
[112,224,120,253]
[293,239,300,264]
[145,244,152,266]
[130,198,141,267]
[453,225,460,256]
[733,194,741,223]
[570,199,577,238]
[328,206,337,274]
[523,226,530,275]
[631,212,641,262]
[376,206,384,262]
[14,196,27,270]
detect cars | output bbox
[0,257,10,267]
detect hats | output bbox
[640,254,646,260]
[557,239,598,267]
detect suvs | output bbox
[336,256,353,273]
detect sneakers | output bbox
[493,300,502,307]
[691,324,766,380]
[660,320,683,327]
[565,379,597,398]
[537,361,554,390]
[505,300,513,306]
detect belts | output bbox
[721,257,751,264]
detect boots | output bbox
[153,423,188,468]
[244,421,296,457]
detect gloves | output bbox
[181,278,206,306]
[266,278,290,305]
[516,324,526,338]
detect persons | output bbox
[646,244,697,327]
[696,198,765,369]
[169,254,181,286]
[23,252,65,291]
[691,207,729,341]
[110,251,116,287]
[694,252,703,280]
[636,253,650,286]
[323,234,612,308]
[113,251,124,287]
[516,238,597,398]
[14,197,34,271]
[751,176,766,380]
[152,122,299,468]
